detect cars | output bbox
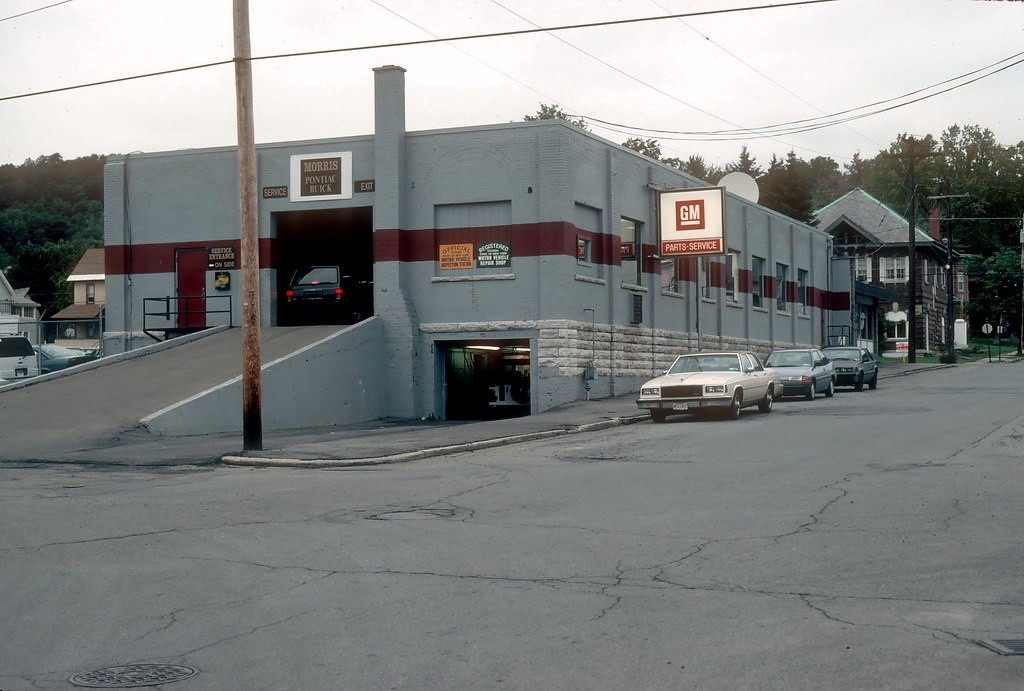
[33,344,104,373]
[762,347,837,401]
[635,351,785,422]
[822,346,879,392]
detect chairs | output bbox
[799,357,811,364]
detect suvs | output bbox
[487,354,531,414]
[0,331,38,388]
[281,265,368,325]
